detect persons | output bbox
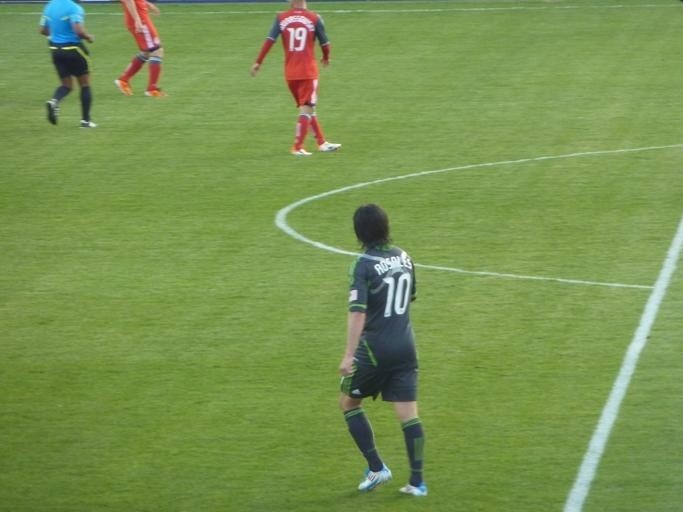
[250,0,342,155]
[340,204,429,497]
[38,0,99,129]
[114,0,168,98]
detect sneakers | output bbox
[46,98,61,126]
[78,119,96,129]
[291,147,313,156]
[398,481,428,498]
[316,140,342,153]
[357,460,392,493]
[113,78,135,96]
[145,88,165,98]
